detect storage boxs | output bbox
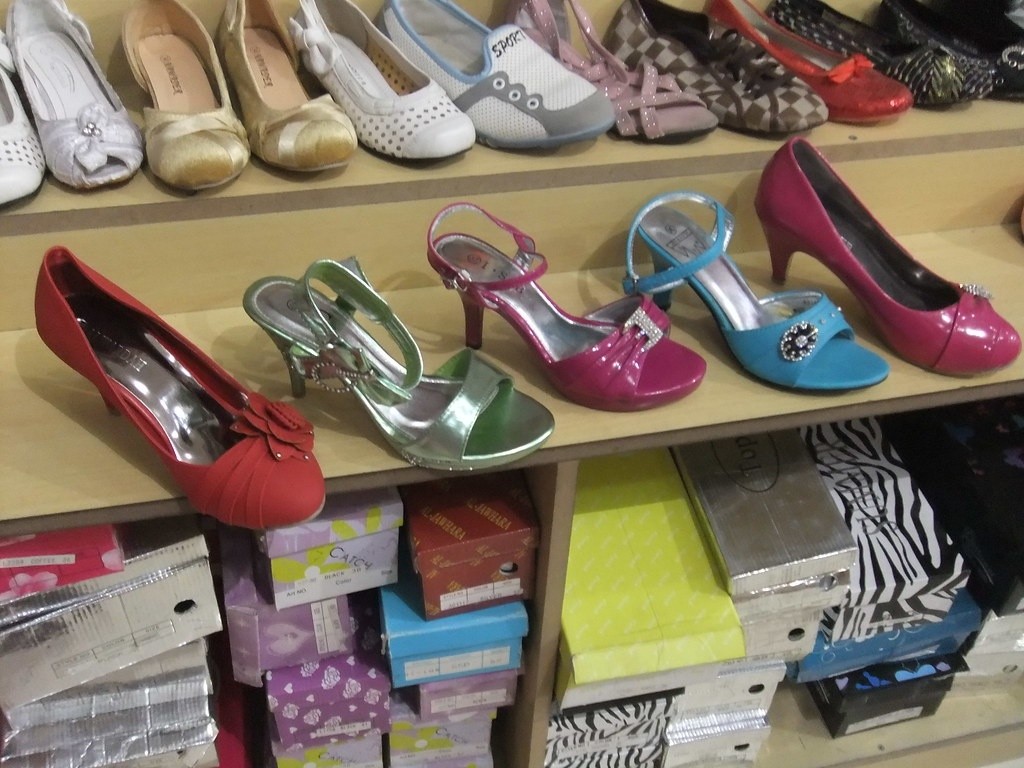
[0,394,1024,768]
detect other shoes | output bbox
[0,0,1024,209]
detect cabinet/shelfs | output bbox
[0,0,1024,768]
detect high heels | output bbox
[426,203,707,412]
[35,245,326,529]
[754,135,1022,377]
[242,256,555,473]
[623,190,889,391]
